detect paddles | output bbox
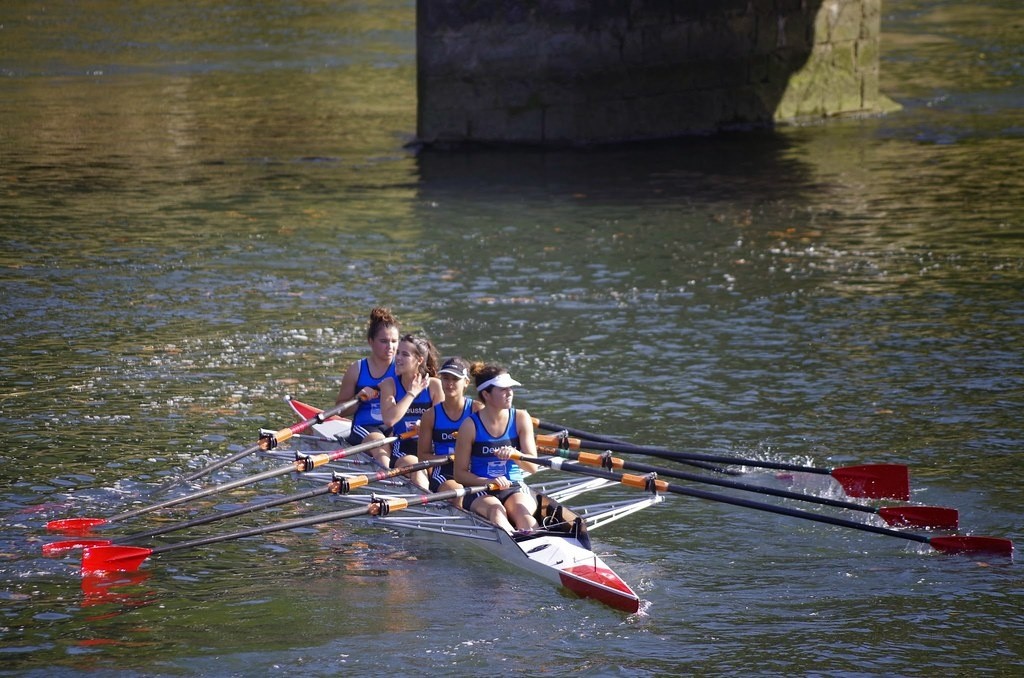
[16,385,378,515]
[41,454,456,558]
[532,445,960,533]
[46,426,420,533]
[530,416,794,480]
[80,480,513,575]
[494,443,1014,568]
[534,429,910,502]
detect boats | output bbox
[259,391,668,617]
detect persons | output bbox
[452,361,539,534]
[419,358,486,509]
[379,334,445,490]
[334,306,402,470]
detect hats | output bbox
[476,374,522,392]
[438,364,470,380]
